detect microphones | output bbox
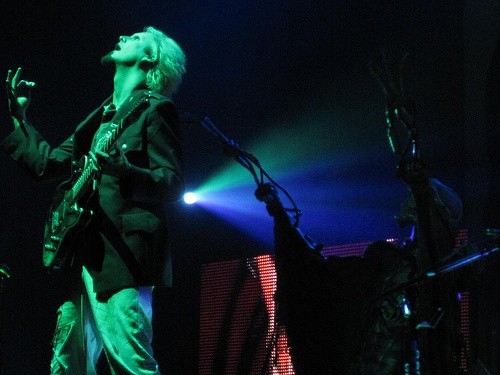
[192,112,256,177]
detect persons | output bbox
[4,27,185,375]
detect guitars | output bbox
[42,98,150,269]
